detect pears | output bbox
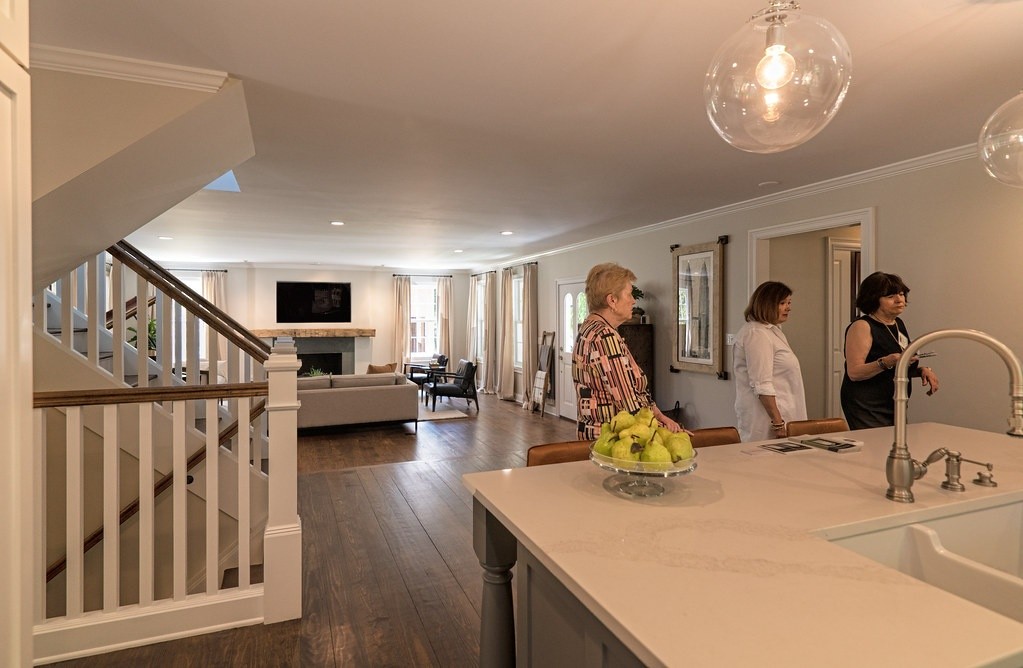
[594,401,693,472]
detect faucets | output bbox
[885,327,1023,503]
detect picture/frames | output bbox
[667,235,732,376]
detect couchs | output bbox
[298,373,420,430]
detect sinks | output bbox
[930,500,1023,584]
[828,528,1023,626]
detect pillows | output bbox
[367,362,400,374]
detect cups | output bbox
[640,315,649,324]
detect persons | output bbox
[840,272,938,431]
[572,261,694,440]
[732,281,807,442]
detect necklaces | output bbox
[591,312,615,330]
[871,312,899,345]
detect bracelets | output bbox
[919,366,931,371]
[770,420,785,431]
[877,358,893,371]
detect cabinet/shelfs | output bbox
[578,321,656,404]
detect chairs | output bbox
[422,359,479,412]
[690,426,739,447]
[403,354,448,384]
[526,439,598,468]
[788,418,848,437]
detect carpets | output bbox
[417,387,468,421]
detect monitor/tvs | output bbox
[276,282,351,323]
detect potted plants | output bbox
[128,315,156,361]
[624,285,645,323]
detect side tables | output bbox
[423,366,450,402]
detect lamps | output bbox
[704,0,856,153]
[979,94,1023,191]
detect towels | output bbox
[531,370,551,405]
[539,345,555,400]
